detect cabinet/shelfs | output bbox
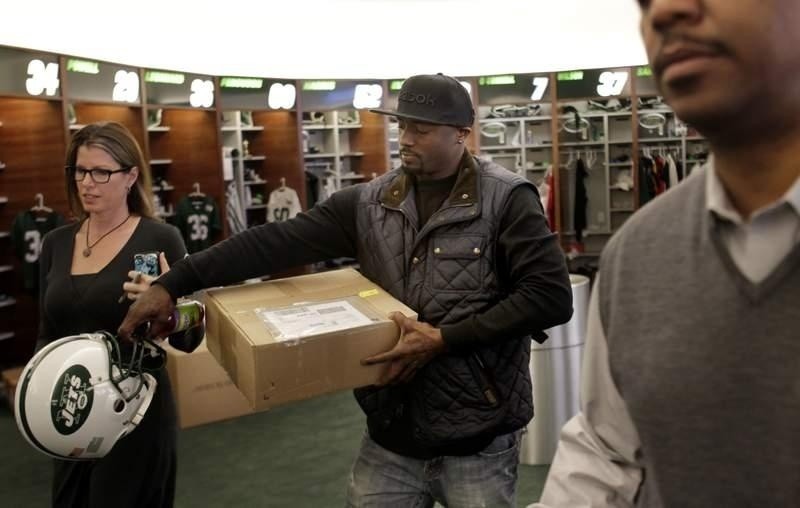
[68,99,144,161]
[474,98,556,227]
[147,105,225,242]
[635,97,711,206]
[300,105,400,209]
[0,99,71,341]
[553,97,635,273]
[222,108,306,239]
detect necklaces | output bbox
[82,215,132,257]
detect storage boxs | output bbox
[205,268,419,411]
[2,368,24,417]
[151,335,273,433]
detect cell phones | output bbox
[131,250,162,277]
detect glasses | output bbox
[64,166,126,184]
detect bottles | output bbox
[134,300,204,343]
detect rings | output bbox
[131,294,136,300]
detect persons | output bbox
[118,72,575,507]
[523,0,800,507]
[33,119,205,507]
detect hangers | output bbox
[186,183,208,199]
[29,193,53,222]
[638,146,682,165]
[272,178,295,193]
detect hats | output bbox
[370,73,475,128]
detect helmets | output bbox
[13,330,168,462]
